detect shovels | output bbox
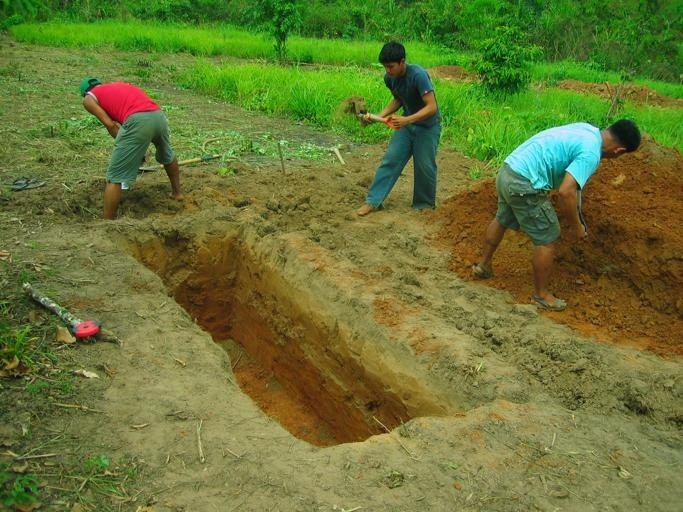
[139,154,222,172]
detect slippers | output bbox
[11,175,32,191]
[24,178,48,189]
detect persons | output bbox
[78,76,183,221]
[353,42,441,218]
[474,118,640,311]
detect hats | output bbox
[80,77,102,95]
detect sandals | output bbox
[529,294,566,311]
[472,264,496,279]
